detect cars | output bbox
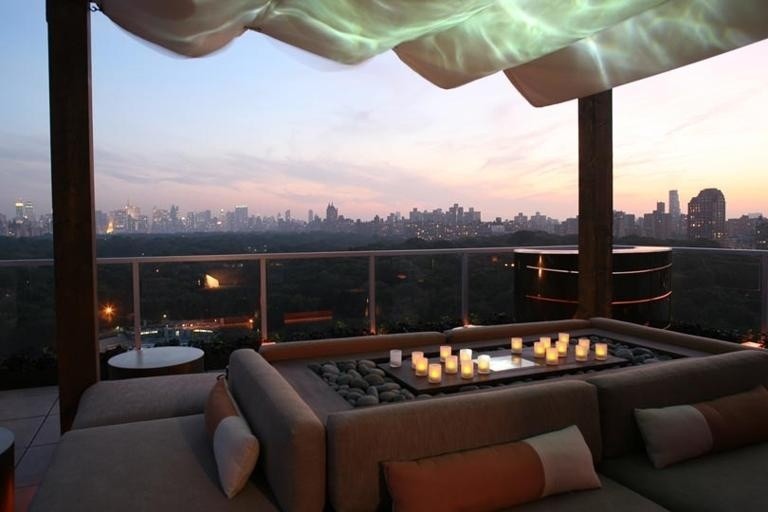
[390,349,402,368]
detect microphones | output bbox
[71,371,225,428]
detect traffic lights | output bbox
[203,378,260,499]
[631,383,767,467]
[378,423,604,512]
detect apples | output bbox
[390,345,491,384]
[511,332,607,366]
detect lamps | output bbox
[325,379,668,511]
[590,350,768,511]
[444,319,591,345]
[592,316,760,353]
[259,331,446,363]
[24,349,327,510]
